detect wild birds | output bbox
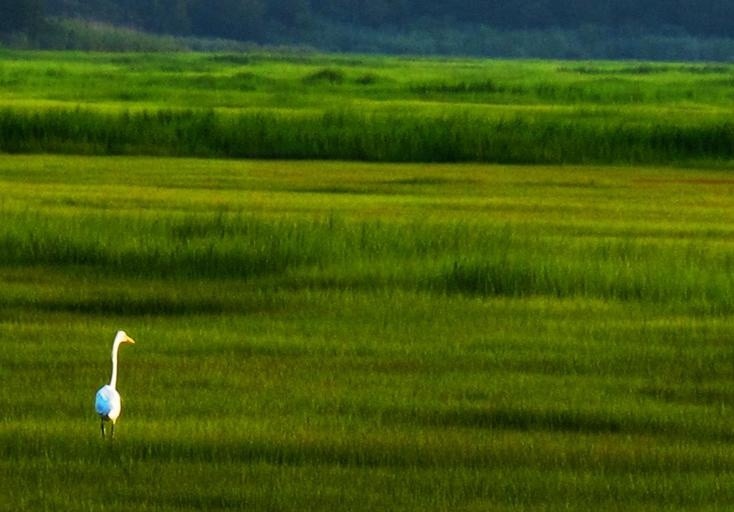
[94,330,136,441]
